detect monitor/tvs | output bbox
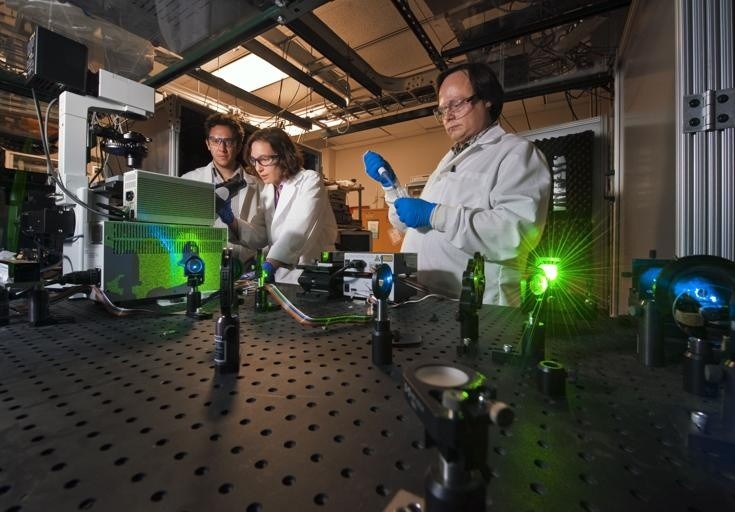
[336,230,371,252]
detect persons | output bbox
[362,63,552,307]
[216,127,338,284]
[180,112,265,271]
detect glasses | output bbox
[206,136,237,147]
[248,154,280,167]
[433,91,481,126]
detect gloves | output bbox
[261,262,276,283]
[363,150,397,187]
[393,197,438,230]
[217,201,234,225]
